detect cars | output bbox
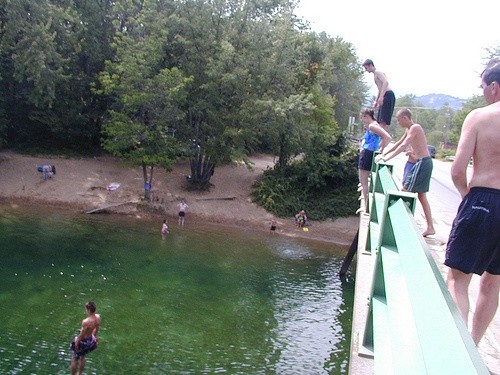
[427,145,436,159]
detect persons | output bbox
[161,219,170,241]
[295,210,307,227]
[269,218,278,232]
[362,59,396,147]
[355,108,393,215]
[177,198,190,227]
[443,58,500,346]
[69,301,101,375]
[374,108,436,237]
